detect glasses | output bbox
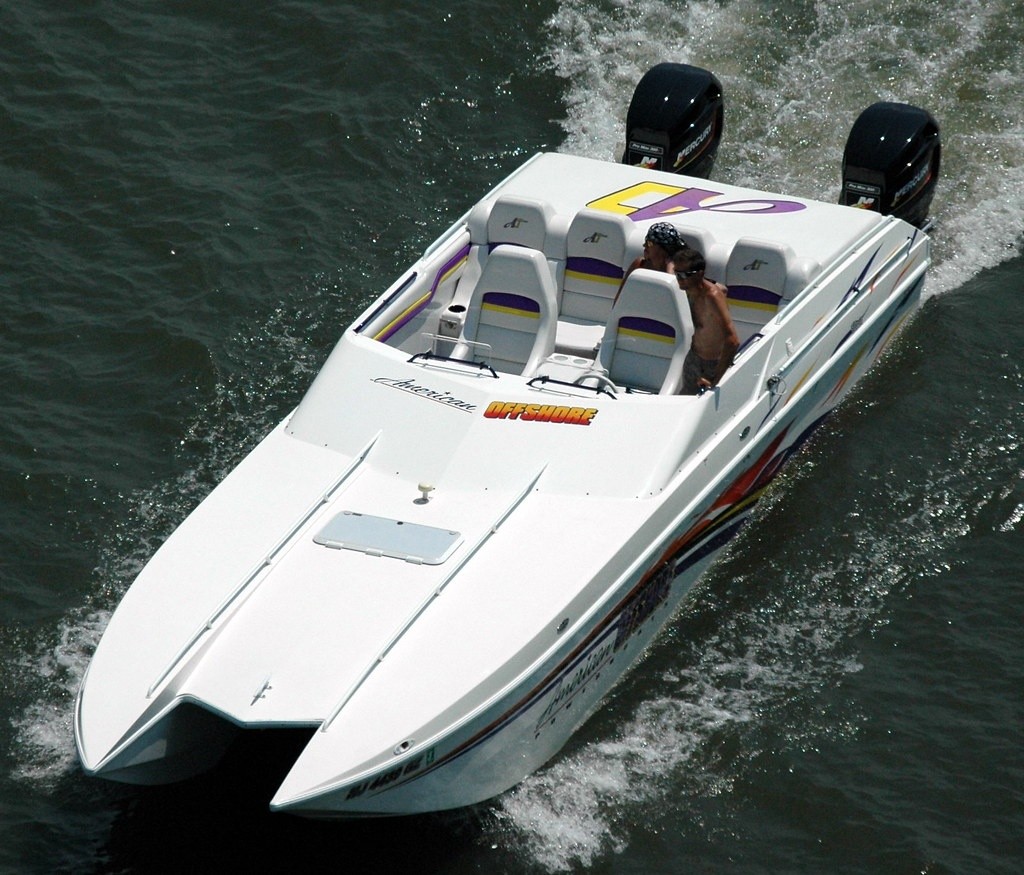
[645,236,661,248]
[674,270,698,280]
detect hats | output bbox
[647,221,685,246]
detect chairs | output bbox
[440,195,806,397]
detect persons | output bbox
[676,248,740,393]
[610,222,689,309]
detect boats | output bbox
[70,61,942,828]
